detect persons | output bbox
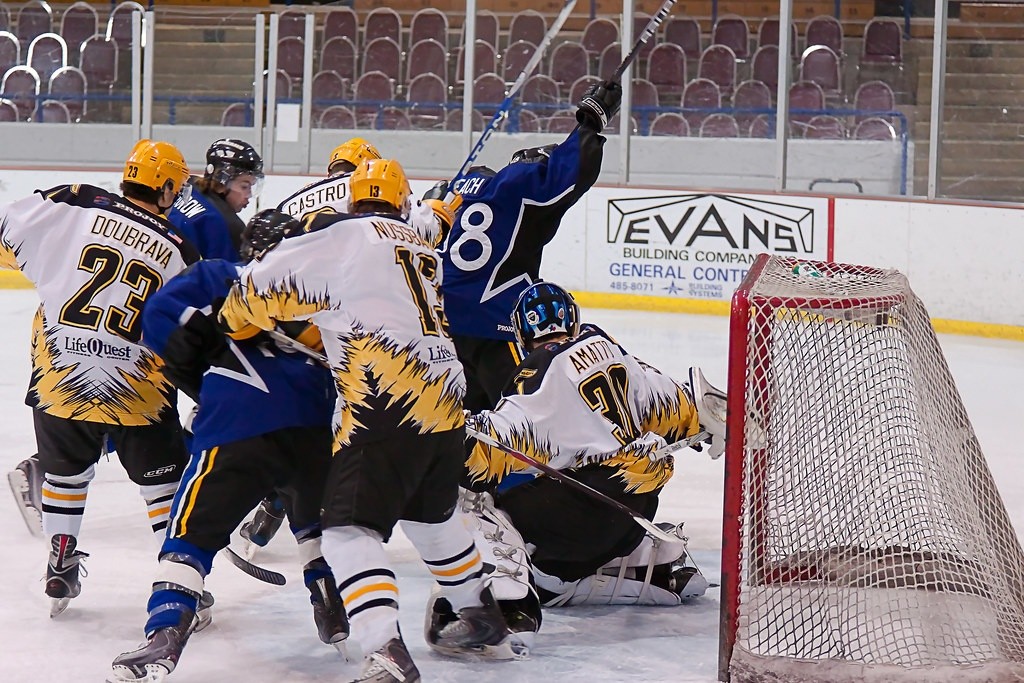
[105,79,763,683]
[5,137,265,537]
[1,140,213,633]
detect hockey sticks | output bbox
[444,0,579,204]
[221,547,289,585]
[651,431,715,462]
[267,327,678,544]
[566,0,679,145]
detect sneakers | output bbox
[347,620,421,683]
[430,569,509,662]
[42,534,90,619]
[104,609,200,683]
[8,457,47,537]
[301,580,350,665]
[237,501,286,563]
[191,593,213,634]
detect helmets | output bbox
[510,278,581,349]
[238,207,304,254]
[347,157,413,208]
[328,137,383,170]
[417,187,464,242]
[205,138,265,179]
[121,138,191,197]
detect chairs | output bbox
[0,0,153,124]
[220,4,904,142]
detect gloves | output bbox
[577,77,623,132]
[508,144,557,168]
